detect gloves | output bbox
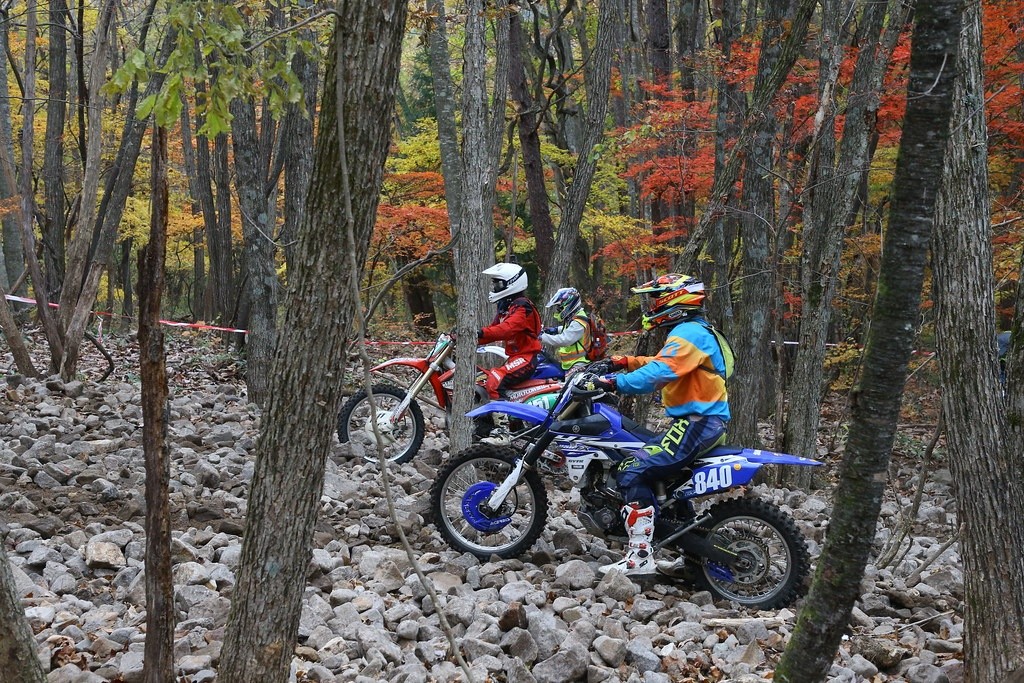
[477,329,483,338]
[574,372,617,391]
[538,332,543,340]
[583,355,627,377]
[544,326,558,334]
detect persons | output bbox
[572,273,729,577]
[539,287,593,374]
[447,261,543,445]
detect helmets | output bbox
[482,262,528,304]
[630,273,708,331]
[545,287,582,322]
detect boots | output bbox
[598,501,659,578]
[479,400,511,447]
[656,500,698,583]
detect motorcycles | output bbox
[426,360,823,611]
[337,329,607,478]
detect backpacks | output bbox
[566,313,608,362]
[664,319,736,388]
[508,297,541,339]
